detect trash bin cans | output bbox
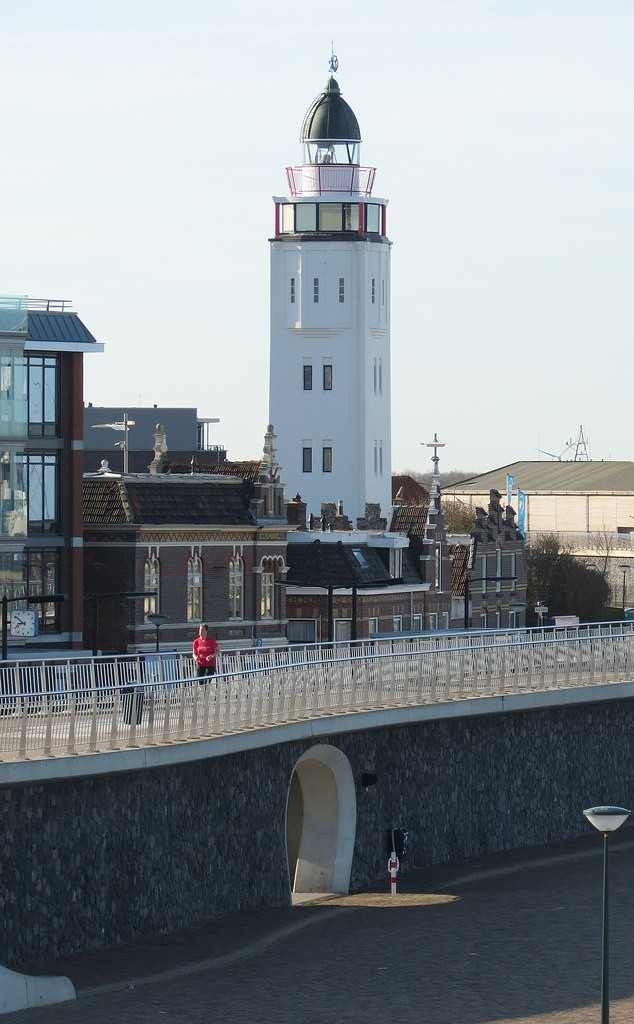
[119,681,145,725]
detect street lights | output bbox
[617,565,631,627]
[0,593,66,693]
[352,573,405,648]
[274,579,389,693]
[117,414,135,473]
[92,591,159,656]
[458,576,519,638]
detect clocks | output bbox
[10,609,38,637]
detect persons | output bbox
[193,625,221,686]
